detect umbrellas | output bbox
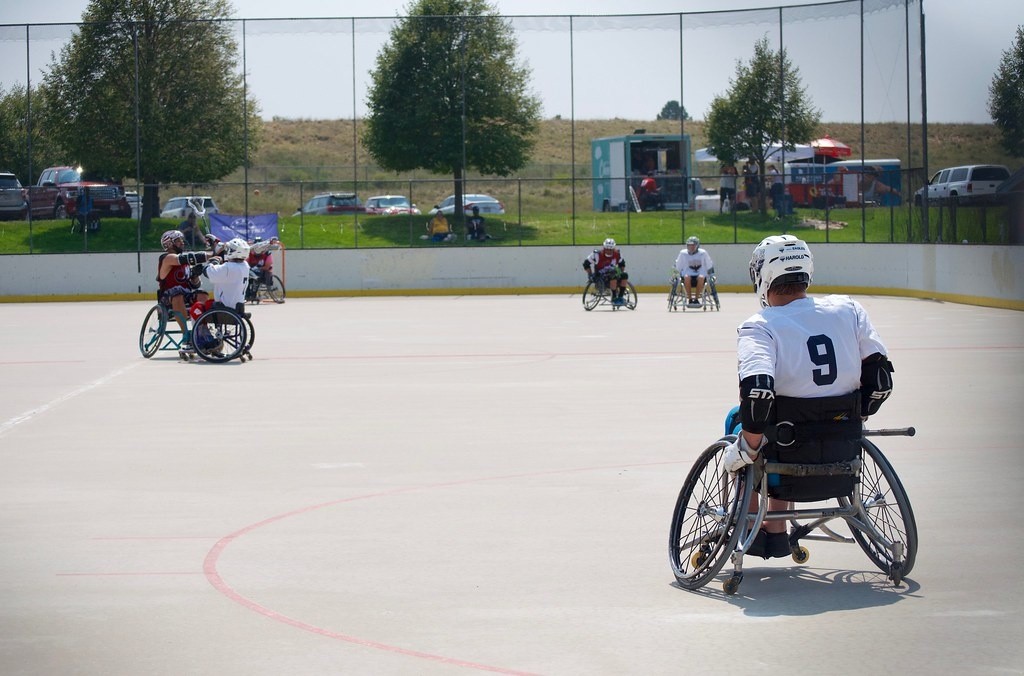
[807,134,851,184]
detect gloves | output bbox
[620,272,628,279]
[588,274,596,281]
[723,432,756,474]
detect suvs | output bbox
[25,166,132,221]
[292,192,366,216]
[913,164,1013,208]
[0,170,27,221]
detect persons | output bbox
[75,185,95,233]
[641,171,663,211]
[671,236,714,308]
[719,160,738,216]
[720,235,895,558]
[427,210,458,243]
[467,206,486,242]
[176,213,209,247]
[156,230,273,353]
[742,158,785,222]
[582,238,628,306]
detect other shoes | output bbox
[244,292,256,302]
[196,337,220,352]
[744,527,767,557]
[611,297,628,306]
[444,234,452,240]
[765,532,793,558]
[694,299,700,304]
[689,299,694,304]
[181,338,196,349]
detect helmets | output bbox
[602,238,616,250]
[749,235,813,309]
[252,236,262,243]
[161,230,184,250]
[223,238,250,260]
[685,236,700,254]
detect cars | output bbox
[429,193,505,217]
[159,195,219,220]
[366,195,421,215]
[124,190,144,219]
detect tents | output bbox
[693,124,815,184]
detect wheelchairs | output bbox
[582,272,637,311]
[667,275,720,312]
[668,385,920,595]
[243,271,287,304]
[139,289,255,364]
